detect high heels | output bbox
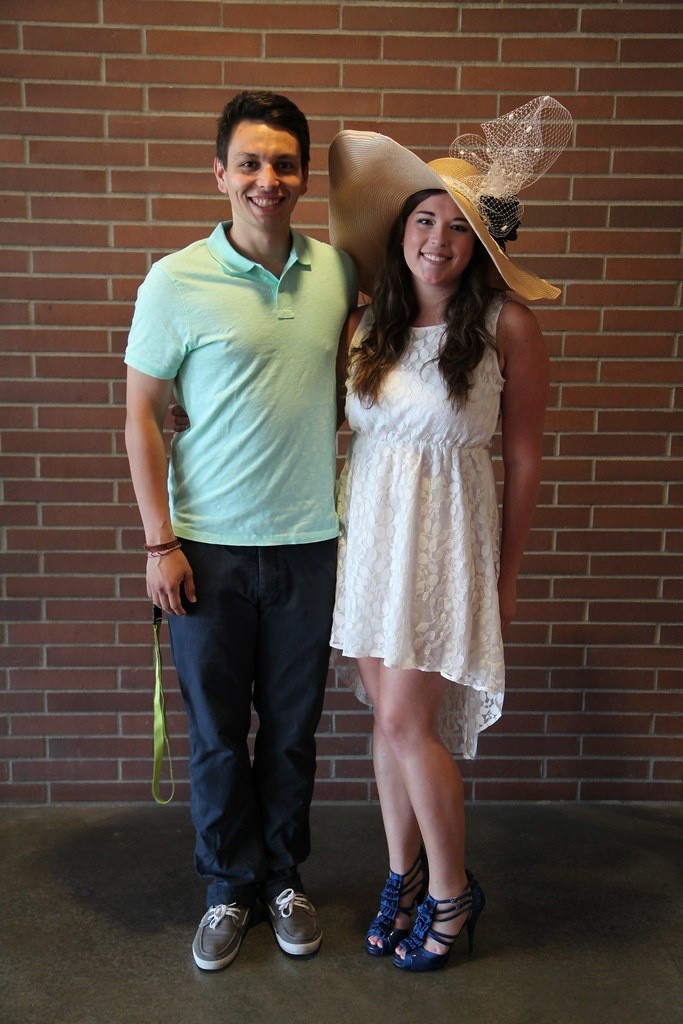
[391,867,485,972]
[364,846,429,958]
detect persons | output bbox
[327,129,561,972]
[124,89,359,970]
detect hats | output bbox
[326,95,577,301]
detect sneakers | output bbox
[191,901,251,969]
[263,890,323,955]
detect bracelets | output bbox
[145,540,181,558]
